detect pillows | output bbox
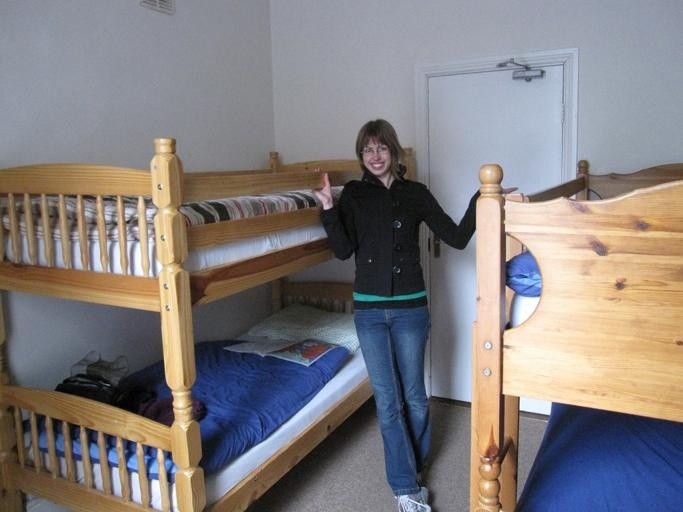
[248,301,361,352]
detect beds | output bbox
[468,160,683,512]
[0,137,417,512]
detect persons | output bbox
[309,118,519,511]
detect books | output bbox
[223,337,337,368]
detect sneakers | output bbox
[396,488,431,512]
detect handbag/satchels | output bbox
[54,374,158,409]
